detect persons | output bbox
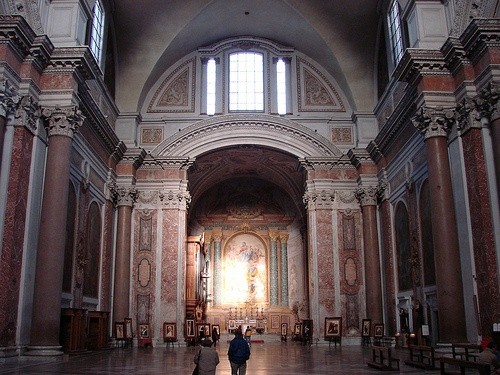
[236,325,242,332]
[192,338,219,375]
[211,328,219,347]
[399,308,407,332]
[199,327,205,348]
[245,326,252,347]
[227,329,250,375]
[304,324,310,346]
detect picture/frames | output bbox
[280,323,288,336]
[374,324,385,339]
[361,319,371,337]
[324,316,342,339]
[137,322,150,339]
[302,319,314,338]
[163,322,178,342]
[115,317,133,340]
[294,323,300,335]
[185,318,220,340]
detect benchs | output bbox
[402,346,441,371]
[440,356,492,375]
[367,345,401,372]
[451,342,485,363]
